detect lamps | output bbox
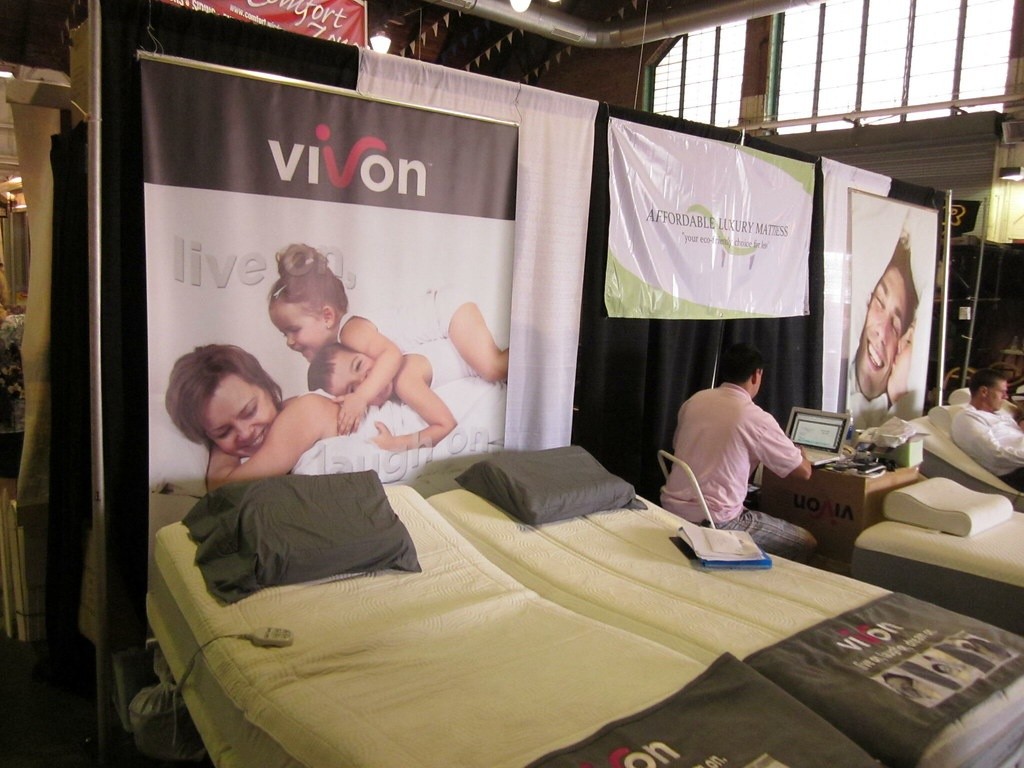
[997,166,1024,182]
[1001,119,1024,145]
[370,22,392,53]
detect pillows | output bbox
[947,387,971,405]
[927,404,969,440]
[181,469,422,606]
[882,476,1014,538]
[453,445,648,525]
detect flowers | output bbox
[0,313,26,400]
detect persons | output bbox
[162,342,506,493]
[267,243,509,436]
[956,636,1009,663]
[925,655,971,684]
[843,233,919,427]
[660,342,819,567]
[883,673,937,707]
[949,368,1024,492]
[305,340,478,452]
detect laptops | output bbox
[785,406,851,465]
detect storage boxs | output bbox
[758,465,918,564]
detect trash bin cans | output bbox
[0,488,48,641]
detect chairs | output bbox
[657,450,716,529]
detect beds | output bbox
[146,485,885,768]
[909,415,1024,513]
[424,489,1024,768]
[849,509,1024,635]
